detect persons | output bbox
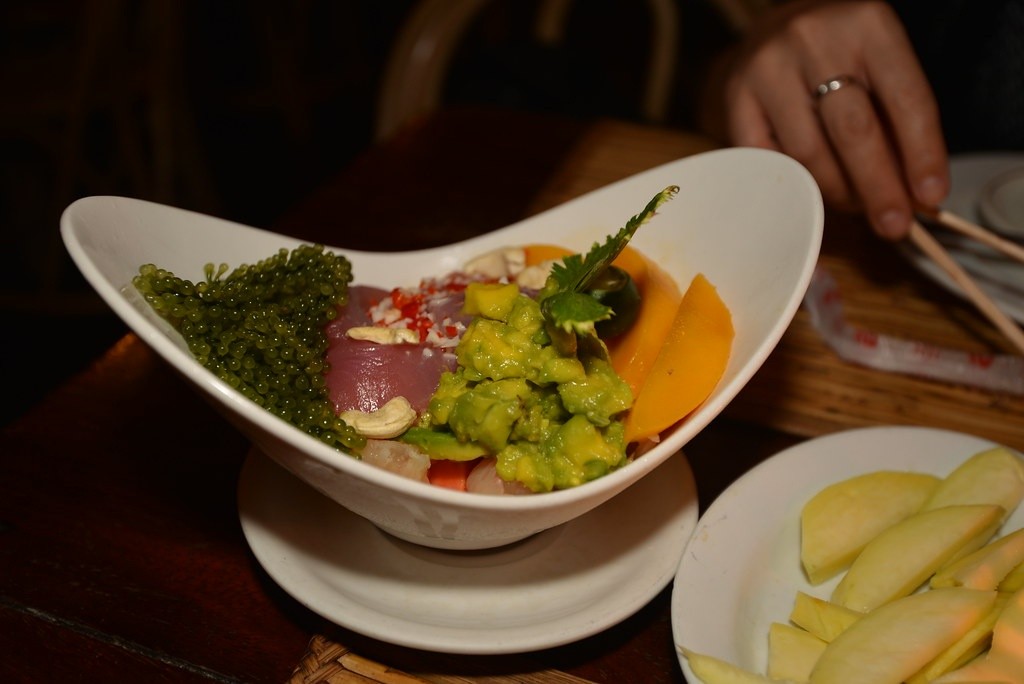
[674,1,951,240]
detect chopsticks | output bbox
[903,204,1024,351]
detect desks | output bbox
[0,107,1024,684]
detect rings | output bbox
[816,72,874,105]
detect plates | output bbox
[236,447,697,653]
[900,155,1024,322]
[670,427,1024,684]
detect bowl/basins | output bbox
[59,147,826,551]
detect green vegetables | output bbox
[541,183,678,334]
